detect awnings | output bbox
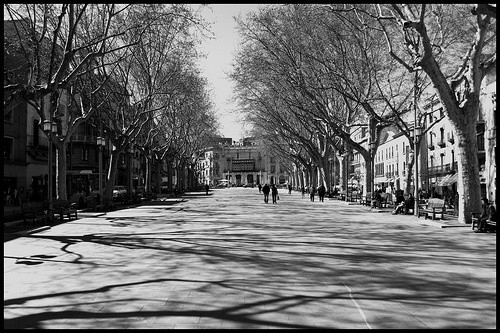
[438,173,459,186]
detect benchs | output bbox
[21,199,79,228]
[87,190,153,212]
[472,211,496,230]
[337,191,447,221]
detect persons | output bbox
[309,186,316,201]
[262,183,271,203]
[272,184,280,204]
[318,184,325,202]
[328,189,338,198]
[258,184,262,193]
[372,187,387,209]
[404,193,415,214]
[288,183,292,194]
[475,197,496,233]
[301,186,305,198]
[205,184,209,194]
